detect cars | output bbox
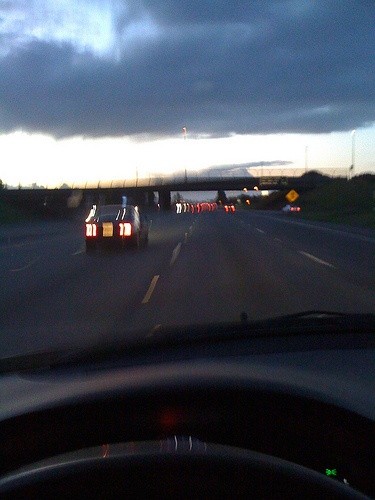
[83,204,151,255]
[282,205,301,216]
